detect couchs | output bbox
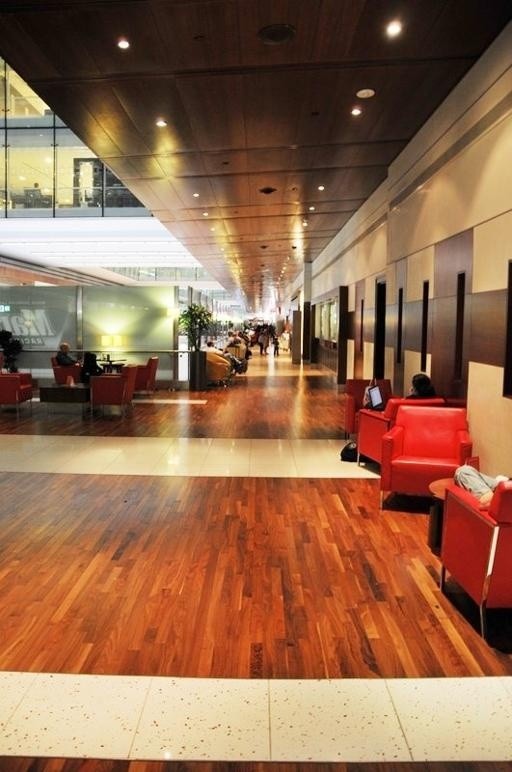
[50,355,82,385]
[377,403,473,512]
[344,377,393,441]
[205,336,248,385]
[0,349,34,422]
[121,356,160,398]
[355,397,446,470]
[88,363,140,424]
[436,475,512,646]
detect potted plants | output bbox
[175,302,218,392]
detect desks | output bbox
[92,359,127,374]
[425,476,506,551]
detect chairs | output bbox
[0,184,138,210]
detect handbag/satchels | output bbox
[363,376,377,406]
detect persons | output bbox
[362,372,437,412]
[455,464,510,508]
[56,342,82,367]
[207,322,282,375]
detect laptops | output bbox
[368,385,388,410]
